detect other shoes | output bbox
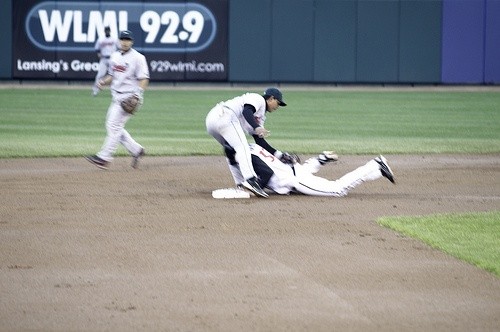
[317,150,339,164]
[238,177,269,199]
[375,154,396,186]
[130,148,145,168]
[81,154,110,170]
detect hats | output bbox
[264,88,288,107]
[118,30,134,40]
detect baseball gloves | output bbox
[119,94,142,115]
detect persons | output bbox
[206,88,287,199]
[92,27,119,95]
[223,142,396,197]
[80,30,150,170]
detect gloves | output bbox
[254,125,271,138]
[279,153,296,165]
